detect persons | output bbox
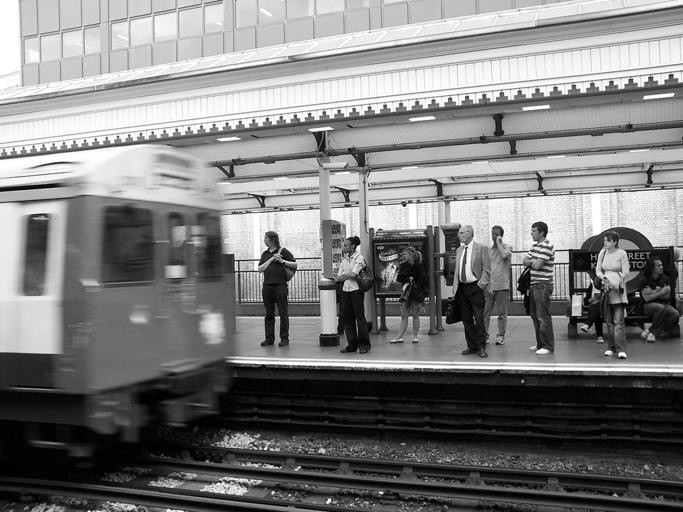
[635,254,680,343]
[580,276,605,342]
[523,222,557,354]
[595,230,630,360]
[483,226,514,344]
[332,236,372,355]
[452,224,490,358]
[389,246,428,344]
[256,231,298,347]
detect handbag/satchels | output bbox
[594,248,607,290]
[277,245,297,282]
[446,295,463,325]
[355,258,374,293]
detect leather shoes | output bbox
[388,337,405,345]
[278,337,290,347]
[411,337,421,345]
[359,345,369,355]
[260,338,274,347]
[479,347,488,358]
[340,346,356,353]
[462,346,477,355]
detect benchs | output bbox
[566,292,682,340]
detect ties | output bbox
[461,246,468,282]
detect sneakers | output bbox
[640,328,657,343]
[580,325,589,333]
[604,349,614,357]
[617,351,628,360]
[496,334,505,345]
[486,333,491,344]
[596,336,605,343]
[529,345,554,355]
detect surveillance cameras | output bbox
[322,162,349,170]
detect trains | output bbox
[0,140,240,472]
